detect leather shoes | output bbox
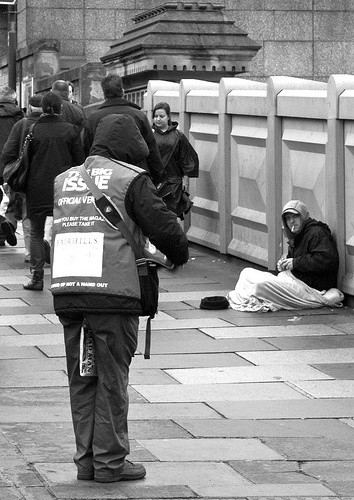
[77,460,146,482]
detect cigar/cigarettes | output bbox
[291,225,295,232]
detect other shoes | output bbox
[44,239,51,264]
[1,221,20,246]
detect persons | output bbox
[0,73,199,291]
[51,113,189,482]
[227,200,345,312]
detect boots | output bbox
[23,270,44,290]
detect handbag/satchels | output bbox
[135,248,174,359]
[3,123,36,190]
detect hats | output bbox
[28,95,44,107]
